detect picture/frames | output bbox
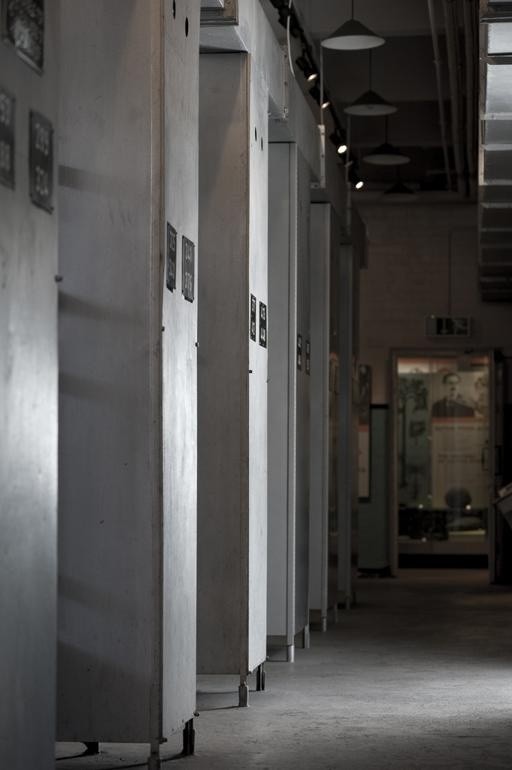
[0,0,45,77]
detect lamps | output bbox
[270,1,409,190]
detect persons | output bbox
[431,373,475,417]
